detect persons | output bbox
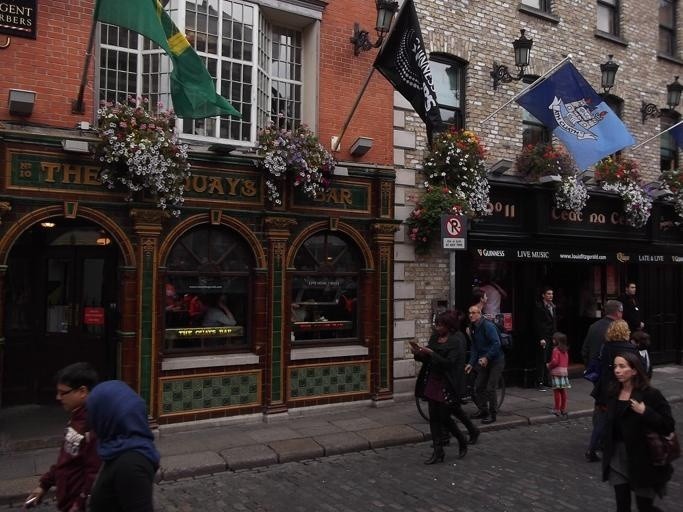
[86,380,161,511]
[163,281,358,347]
[580,282,652,462]
[410,270,510,464]
[546,332,571,414]
[22,362,100,511]
[532,287,560,391]
[593,352,683,512]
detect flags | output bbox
[374,1,440,146]
[95,0,243,124]
[517,61,639,174]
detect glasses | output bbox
[55,388,76,396]
[466,311,479,316]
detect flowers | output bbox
[73,90,192,224]
[398,122,494,259]
[238,106,337,210]
[507,137,682,229]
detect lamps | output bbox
[487,28,533,92]
[638,74,682,127]
[347,134,374,158]
[595,52,619,108]
[6,87,36,119]
[58,139,91,154]
[347,0,401,60]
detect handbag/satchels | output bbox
[639,385,681,469]
[583,339,605,385]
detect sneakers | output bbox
[583,448,599,463]
[535,378,552,393]
[551,407,569,417]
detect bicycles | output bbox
[411,356,510,422]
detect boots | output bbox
[423,423,446,466]
[429,429,451,448]
[447,422,472,459]
[463,419,481,446]
[471,394,490,419]
[481,393,497,424]
